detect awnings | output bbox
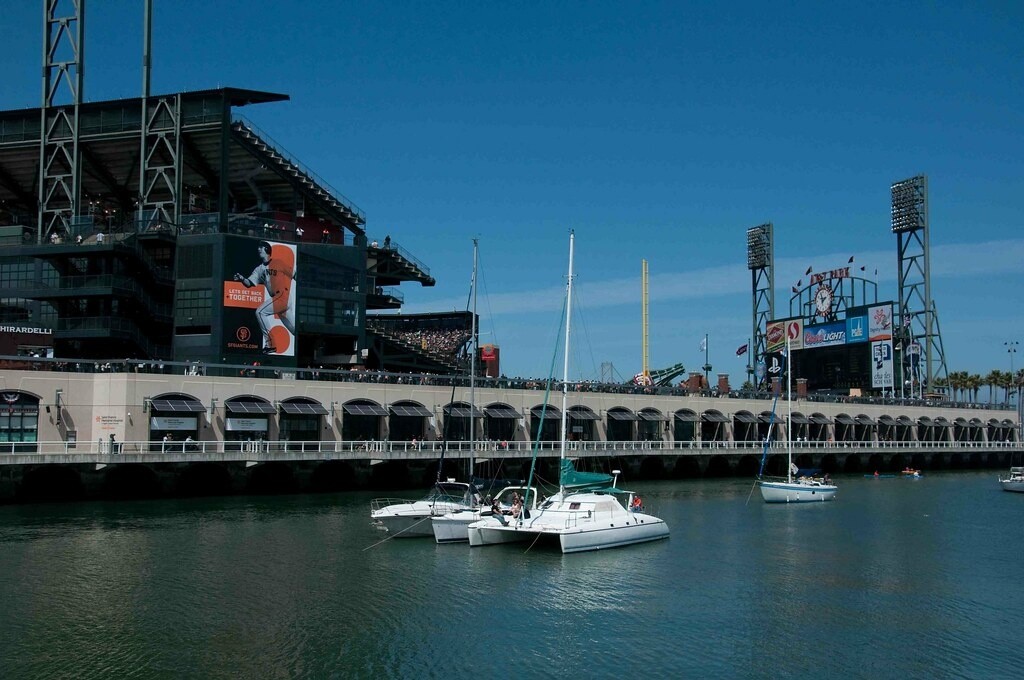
[151,400,1020,429]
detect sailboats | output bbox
[427,237,545,546]
[756,333,838,503]
[469,230,669,555]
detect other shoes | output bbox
[504,521,509,526]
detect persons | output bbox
[632,495,643,513]
[321,229,329,244]
[874,469,879,476]
[234,242,296,354]
[790,463,831,485]
[96,231,104,245]
[384,235,391,249]
[408,433,509,450]
[263,223,305,241]
[914,470,921,478]
[76,234,82,245]
[51,232,58,243]
[28,326,1009,411]
[753,434,895,448]
[491,489,530,527]
[372,239,379,248]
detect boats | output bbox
[863,474,895,478]
[369,475,489,541]
[998,467,1024,493]
[901,469,926,480]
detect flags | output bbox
[847,255,878,275]
[792,266,812,294]
[698,338,706,352]
[736,344,748,357]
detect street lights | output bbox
[1004,341,1019,398]
